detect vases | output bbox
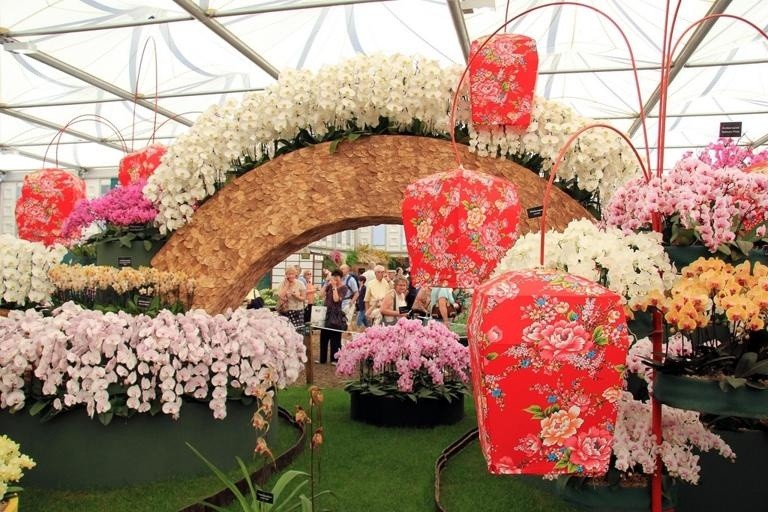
[1,493,17,512]
[349,389,464,428]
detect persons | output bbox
[248,265,474,365]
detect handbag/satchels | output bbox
[432,299,457,318]
[279,299,288,314]
[311,306,327,323]
[326,307,347,330]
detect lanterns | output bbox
[14,145,167,246]
[468,265,629,477]
[402,169,521,287]
[469,34,538,131]
[739,159,768,229]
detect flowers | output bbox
[1,182,309,419]
[141,49,649,236]
[330,315,477,404]
[186,358,341,511]
[487,130,768,485]
[0,432,35,507]
